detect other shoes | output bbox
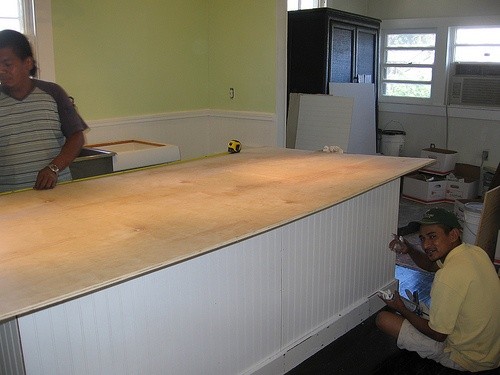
[419,301,430,320]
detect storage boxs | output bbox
[420,147,458,176]
[446,163,481,201]
[403,173,446,204]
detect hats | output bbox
[408,208,459,229]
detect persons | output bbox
[0,29,89,194]
[375,208,500,373]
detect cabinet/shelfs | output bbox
[287,7,381,123]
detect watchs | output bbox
[47,162,60,177]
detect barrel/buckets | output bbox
[377,121,406,157]
[493,229,500,266]
[482,172,495,199]
[462,203,483,246]
